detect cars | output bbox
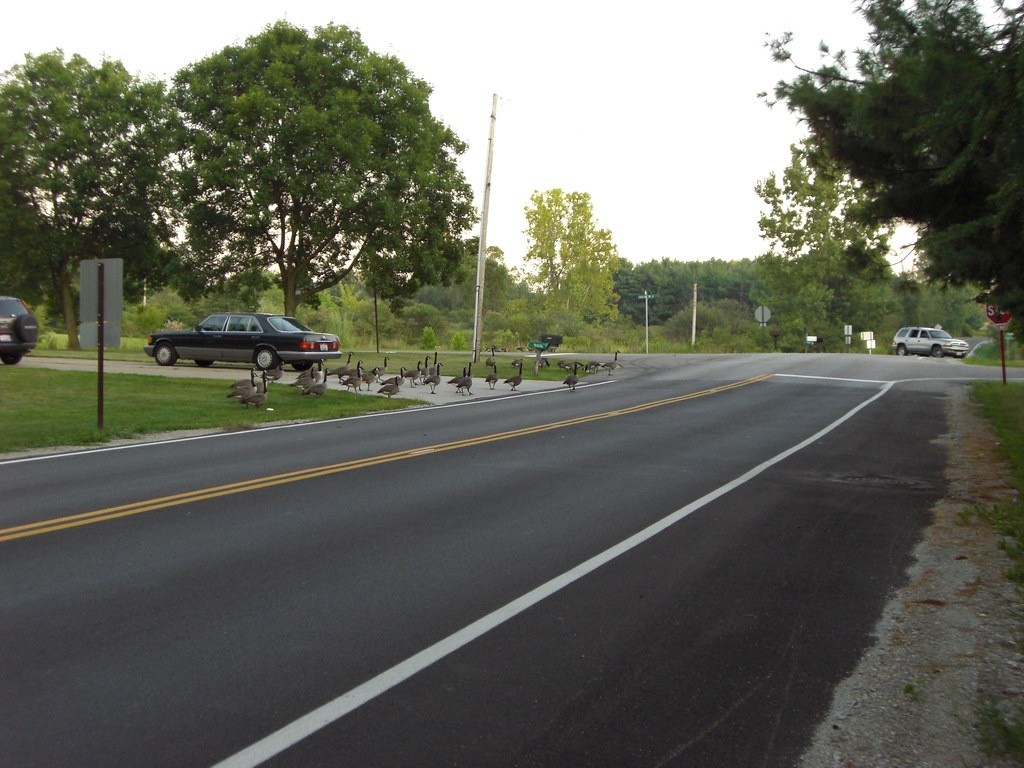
[141,312,343,369]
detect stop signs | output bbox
[986,302,1015,326]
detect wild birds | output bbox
[226,345,621,409]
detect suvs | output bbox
[892,326,969,358]
[0,295,41,365]
